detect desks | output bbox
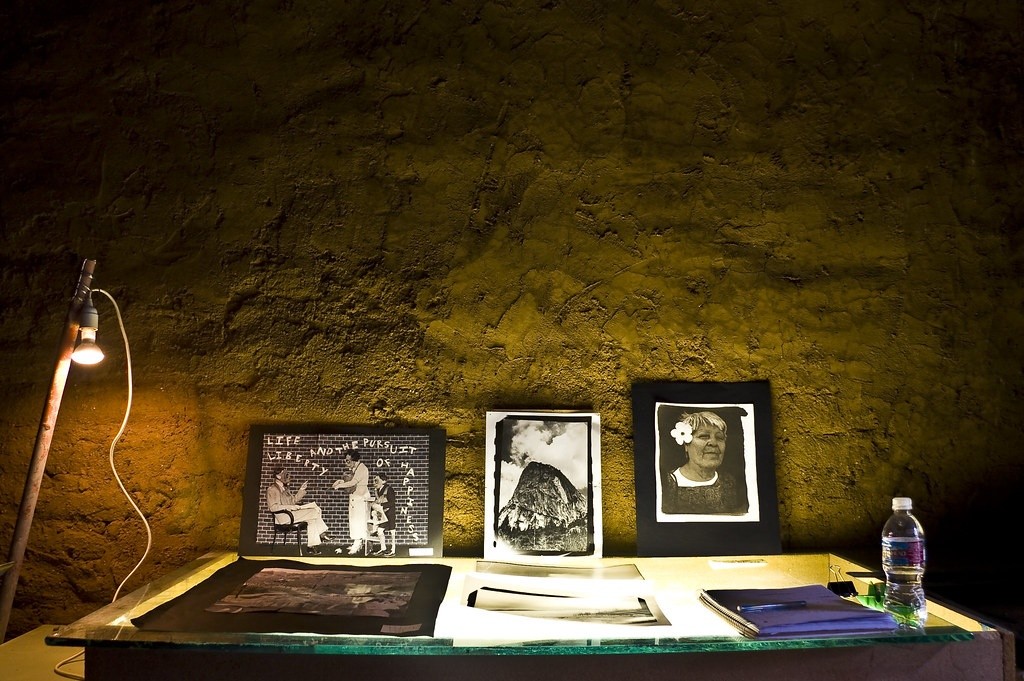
[44,551,1016,681]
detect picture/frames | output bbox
[237,423,446,560]
[632,380,781,559]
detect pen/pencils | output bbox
[737,600,807,613]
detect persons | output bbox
[660,410,750,514]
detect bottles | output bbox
[881,497,927,629]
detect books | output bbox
[702,585,896,636]
